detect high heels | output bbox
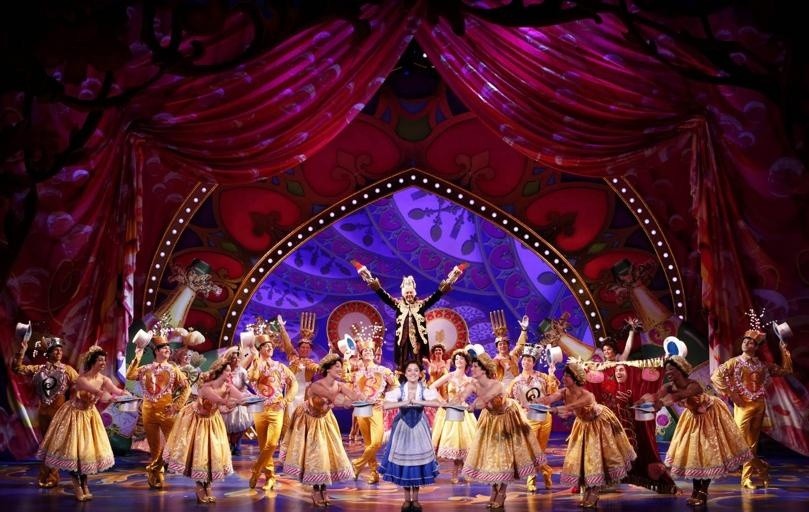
[577,492,600,510]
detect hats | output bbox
[526,402,552,422]
[14,320,33,343]
[451,343,496,378]
[629,401,657,422]
[242,397,266,414]
[441,403,469,422]
[113,395,143,413]
[83,345,105,372]
[207,330,254,378]
[318,332,376,375]
[132,328,173,348]
[743,329,764,345]
[350,400,376,417]
[662,335,695,377]
[254,333,273,350]
[44,336,63,349]
[489,308,511,343]
[770,320,793,343]
[520,342,542,360]
[546,346,587,386]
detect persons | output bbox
[360,266,460,382]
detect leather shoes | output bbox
[526,461,553,492]
[686,489,709,507]
[37,469,59,489]
[451,469,472,485]
[400,499,424,512]
[72,485,94,503]
[745,463,769,491]
[310,491,336,507]
[485,488,506,510]
[145,462,166,490]
[350,457,380,485]
[249,465,275,491]
[195,487,217,505]
